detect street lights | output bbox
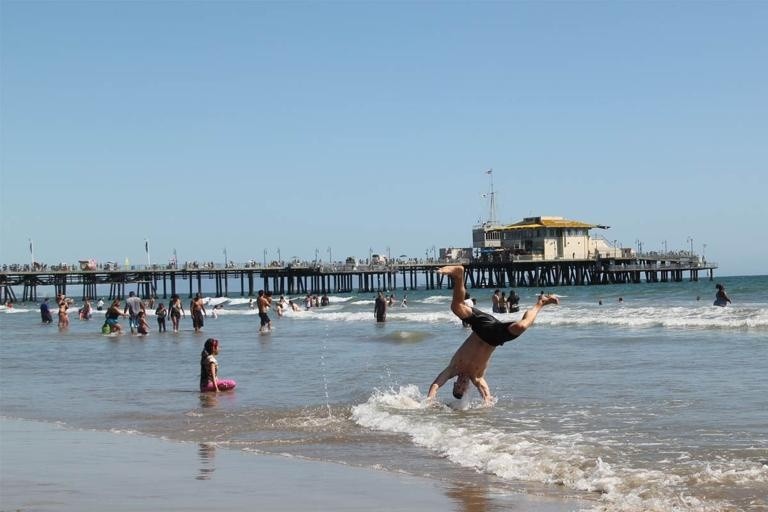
[262,247,267,266]
[424,248,429,259]
[685,235,694,255]
[172,248,177,267]
[314,247,319,261]
[220,247,227,266]
[430,245,437,262]
[659,240,667,254]
[369,247,374,262]
[275,245,281,262]
[385,247,390,258]
[325,245,331,263]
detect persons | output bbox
[40,294,105,331]
[499,292,508,313]
[619,297,623,303]
[212,305,224,319]
[303,291,329,311]
[462,292,473,328]
[472,299,476,305]
[373,289,408,322]
[714,284,731,306]
[492,290,500,313]
[6,301,13,311]
[541,291,544,296]
[427,265,559,403]
[200,339,236,393]
[599,300,602,305]
[250,289,301,332]
[102,291,207,336]
[507,290,520,313]
